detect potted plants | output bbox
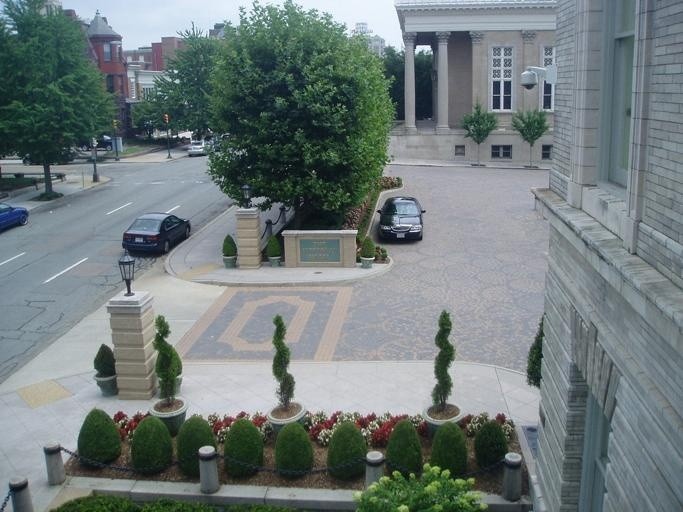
[93,343,117,396]
[267,314,305,434]
[222,235,238,269]
[422,309,463,435]
[267,235,280,266]
[360,236,375,268]
[149,314,187,438]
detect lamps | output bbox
[240,180,252,208]
[117,243,136,296]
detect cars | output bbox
[0,203,29,231]
[23,147,75,166]
[188,140,221,156]
[122,213,191,254]
[376,196,427,242]
[81,135,112,152]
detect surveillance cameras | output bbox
[520,70,538,89]
